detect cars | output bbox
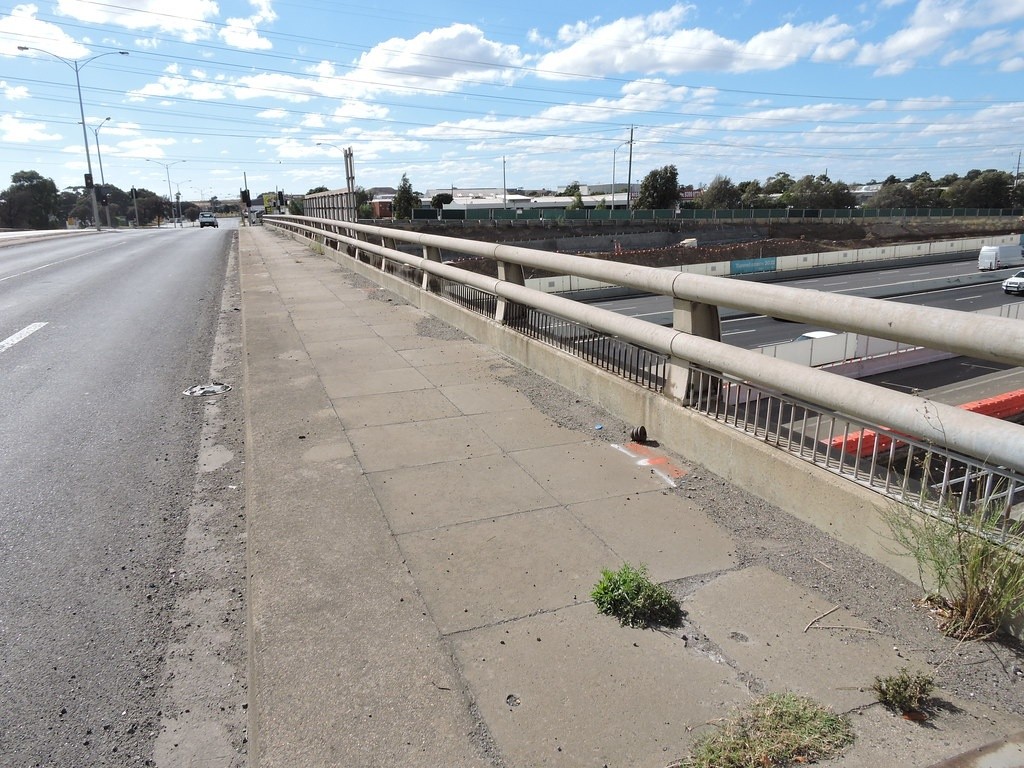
[243,209,264,224]
[789,330,838,343]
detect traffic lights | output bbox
[100,194,107,207]
[85,174,94,188]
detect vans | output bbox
[977,244,1024,273]
[199,211,219,228]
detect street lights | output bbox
[162,179,191,226]
[77,117,111,229]
[15,46,129,232]
[315,143,352,219]
[612,141,636,210]
[189,186,212,200]
[146,159,186,228]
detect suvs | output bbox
[1002,269,1024,297]
